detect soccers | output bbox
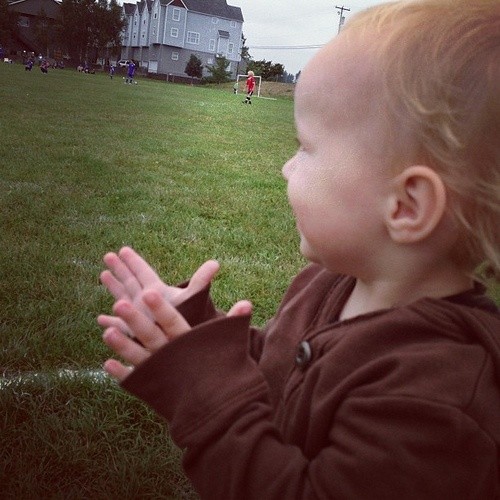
[135,81,138,85]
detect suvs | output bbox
[117,59,135,68]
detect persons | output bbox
[98,1,500,500]
[84,64,90,73]
[110,65,115,79]
[4,53,65,78]
[233,82,239,95]
[242,71,256,104]
[124,59,136,85]
[77,63,84,73]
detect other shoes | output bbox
[242,100,251,104]
